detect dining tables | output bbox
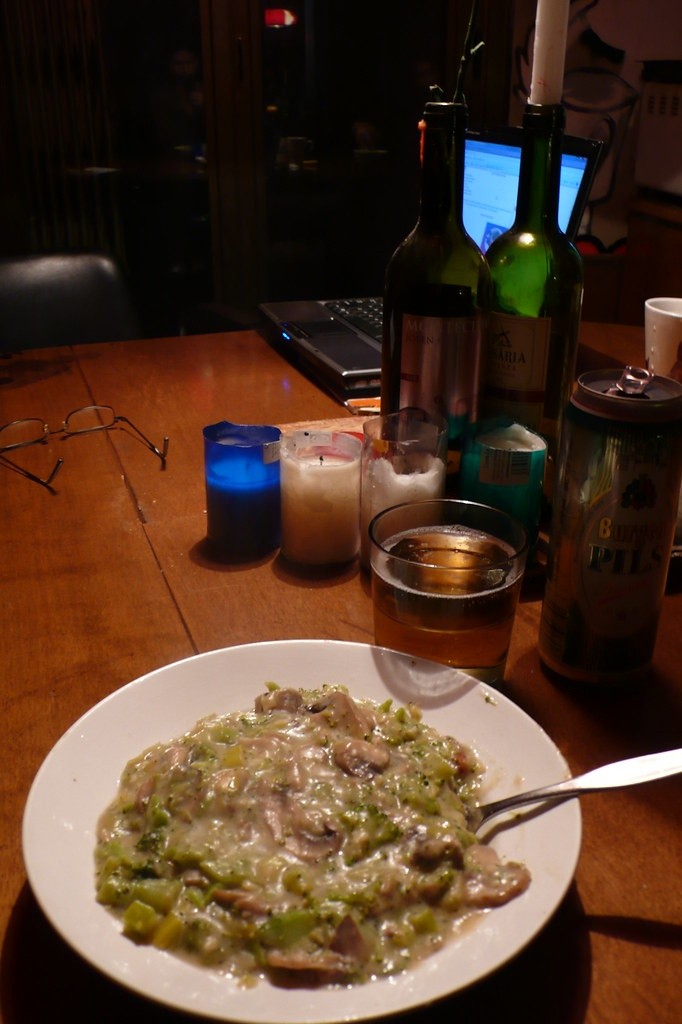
[1,329,682,1024]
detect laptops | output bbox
[260,116,603,393]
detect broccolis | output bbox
[92,680,475,965]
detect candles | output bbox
[201,416,547,580]
[645,295,682,386]
[528,0,570,105]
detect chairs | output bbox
[0,251,139,355]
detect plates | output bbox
[21,639,583,1024]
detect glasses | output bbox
[0,406,169,486]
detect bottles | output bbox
[379,98,585,555]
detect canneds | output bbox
[535,363,682,688]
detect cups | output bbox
[460,419,548,554]
[645,297,682,385]
[367,497,528,687]
